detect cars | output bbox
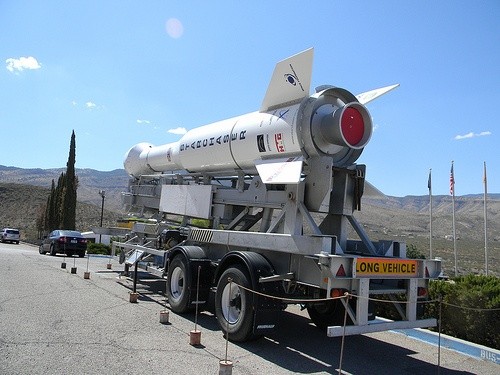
[0,228,20,244]
[39,230,87,258]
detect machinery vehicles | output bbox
[112,47,449,343]
